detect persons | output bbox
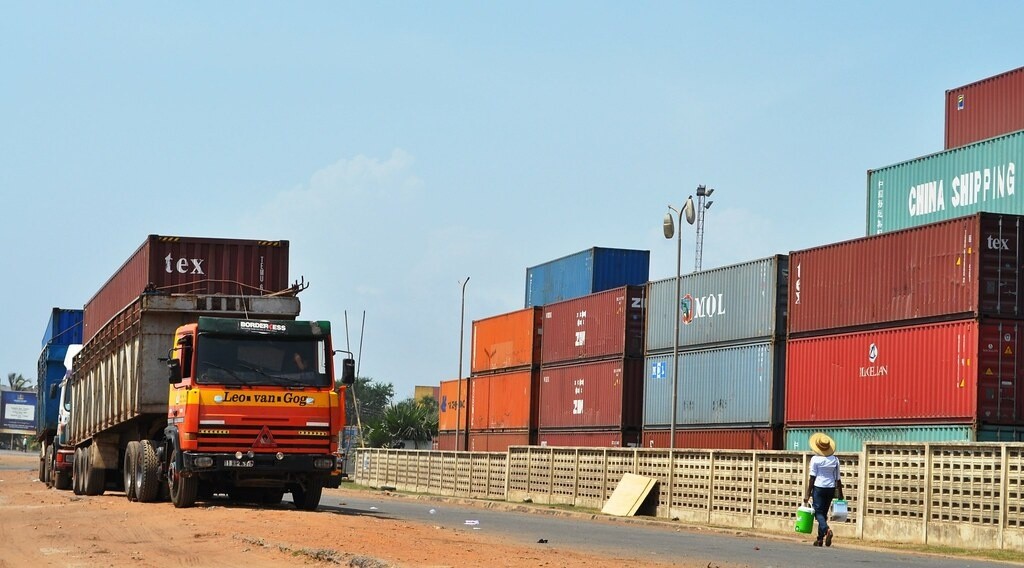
[285,350,311,376]
[21,436,28,453]
[803,432,844,546]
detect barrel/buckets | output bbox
[795,501,815,534]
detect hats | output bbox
[810,432,836,457]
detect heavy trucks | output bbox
[32,234,356,511]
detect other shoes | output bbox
[825,527,833,546]
[813,537,823,546]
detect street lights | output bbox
[453,276,474,452]
[660,195,698,521]
[483,348,497,451]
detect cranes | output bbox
[695,183,715,271]
